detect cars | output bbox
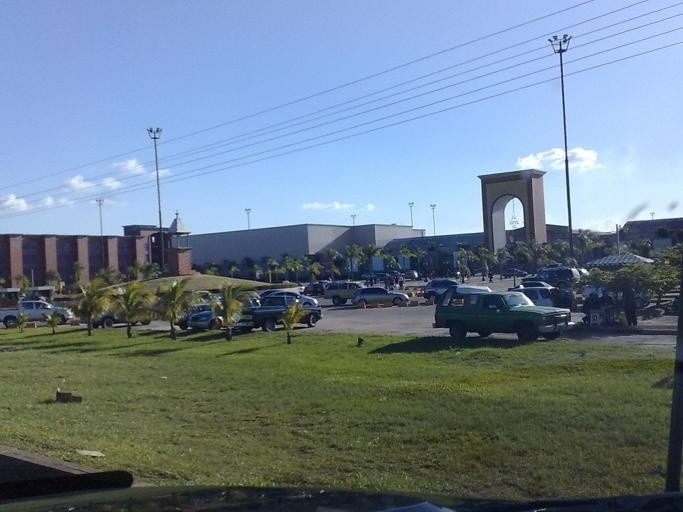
[93,308,150,328]
[424,264,590,340]
[175,270,418,330]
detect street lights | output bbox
[350,213,357,226]
[650,212,655,220]
[548,34,574,258]
[96,199,104,236]
[146,126,165,277]
[408,201,414,226]
[430,203,437,236]
[244,208,251,230]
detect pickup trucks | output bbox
[0,301,75,327]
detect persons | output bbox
[366,277,374,287]
[298,285,305,295]
[384,270,405,291]
[28,289,44,309]
[582,282,638,329]
